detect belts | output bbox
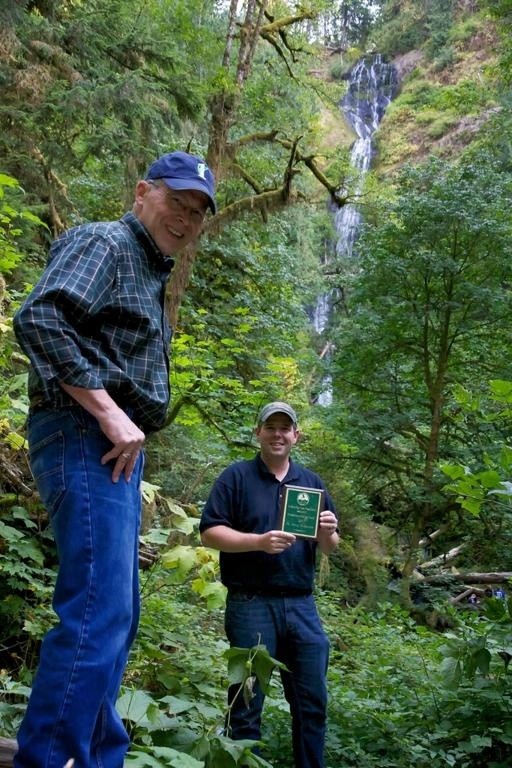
[228,588,313,598]
[28,407,146,435]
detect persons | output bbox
[13,152,216,768]
[199,402,339,768]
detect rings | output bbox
[121,453,132,459]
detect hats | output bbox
[143,151,219,216]
[257,399,299,429]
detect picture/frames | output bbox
[275,481,323,544]
